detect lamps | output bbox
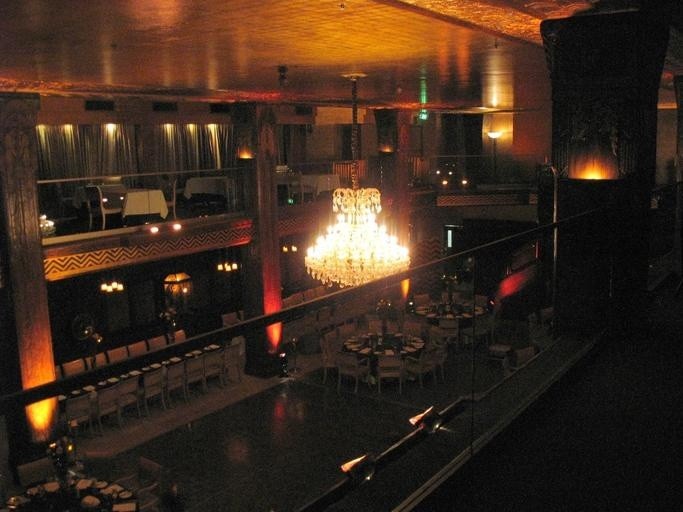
[304,73,412,290]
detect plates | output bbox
[58,341,220,401]
[7,478,134,512]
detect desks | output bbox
[300,173,340,200]
[185,176,230,212]
[72,184,126,209]
[120,189,168,226]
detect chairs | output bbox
[56,313,252,442]
[3,447,179,512]
[278,284,500,399]
[164,178,178,220]
[287,171,315,203]
[84,185,123,232]
